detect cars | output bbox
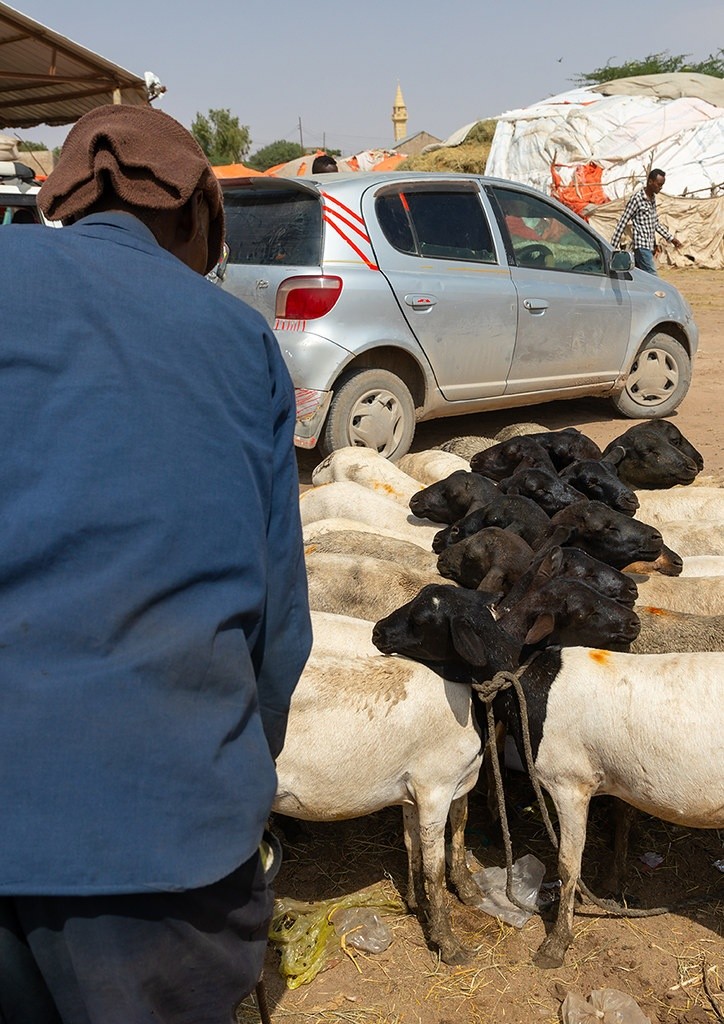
[0,185,64,229]
[206,172,699,461]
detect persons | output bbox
[312,155,339,175]
[610,168,683,278]
[0,99,315,1024]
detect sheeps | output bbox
[271,412,723,970]
